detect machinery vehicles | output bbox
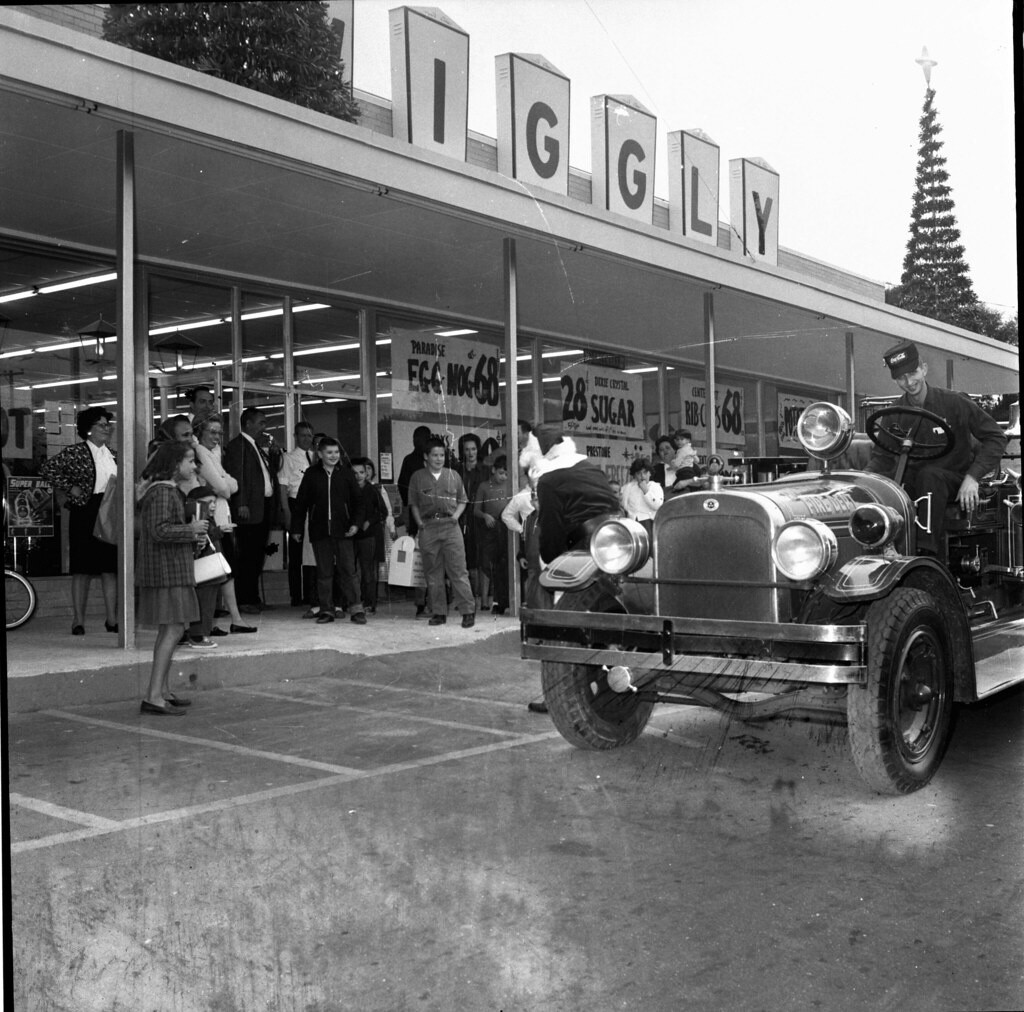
[518,402,1024,796]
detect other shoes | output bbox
[290,594,504,628]
[210,627,227,636]
[257,602,274,611]
[230,624,257,633]
[241,604,259,614]
[71,625,85,635]
[104,621,119,632]
[528,702,548,712]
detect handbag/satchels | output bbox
[92,474,118,545]
[193,534,232,584]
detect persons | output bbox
[609,428,699,530]
[38,406,119,636]
[519,423,623,713]
[865,342,1008,554]
[159,387,535,649]
[134,440,209,716]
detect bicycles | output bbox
[3,570,35,630]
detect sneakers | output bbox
[181,637,217,648]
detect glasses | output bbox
[205,429,225,437]
[92,423,110,429]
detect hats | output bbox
[186,486,218,500]
[883,341,919,379]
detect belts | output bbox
[422,513,450,519]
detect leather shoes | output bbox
[164,694,191,706]
[141,699,186,715]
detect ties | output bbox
[254,441,272,485]
[306,452,312,467]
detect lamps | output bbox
[78,313,116,372]
[0,268,331,358]
[15,326,479,391]
[149,332,204,374]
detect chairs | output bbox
[943,460,1001,530]
